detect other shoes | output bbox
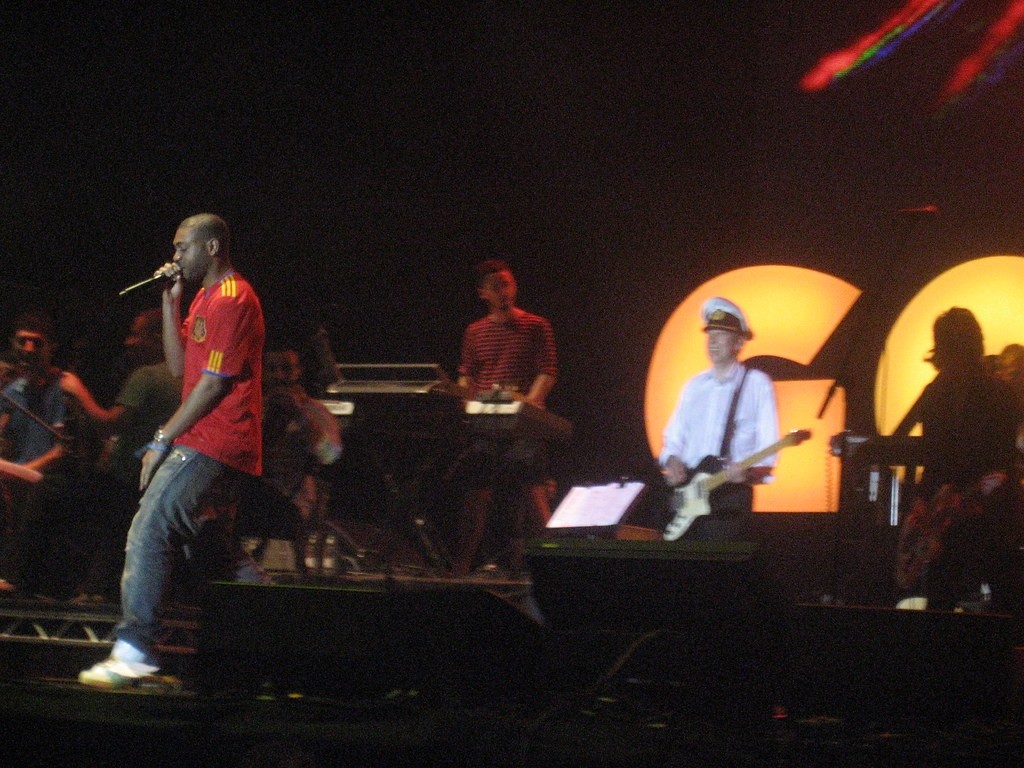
[78,658,141,691]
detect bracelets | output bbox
[148,430,172,453]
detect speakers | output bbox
[782,601,1018,721]
[193,581,560,706]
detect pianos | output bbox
[308,360,578,449]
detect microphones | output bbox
[120,266,183,297]
[899,205,939,216]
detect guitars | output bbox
[643,427,813,542]
[892,453,1024,589]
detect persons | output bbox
[659,297,780,511]
[889,307,1024,517]
[0,211,343,689]
[542,473,560,510]
[448,260,558,575]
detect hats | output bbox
[702,296,750,339]
